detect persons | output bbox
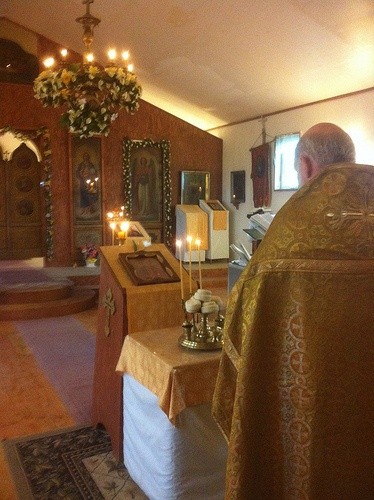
[295,122,355,188]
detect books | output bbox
[230,242,251,264]
[250,213,274,231]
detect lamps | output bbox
[42,0,136,74]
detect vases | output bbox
[85,257,97,267]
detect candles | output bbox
[110,221,129,246]
[175,236,202,299]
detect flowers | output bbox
[32,63,143,139]
[78,241,100,263]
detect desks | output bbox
[114,324,231,500]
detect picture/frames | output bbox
[180,170,211,208]
[67,133,106,267]
[130,143,164,244]
[231,170,245,204]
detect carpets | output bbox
[0,420,148,500]
